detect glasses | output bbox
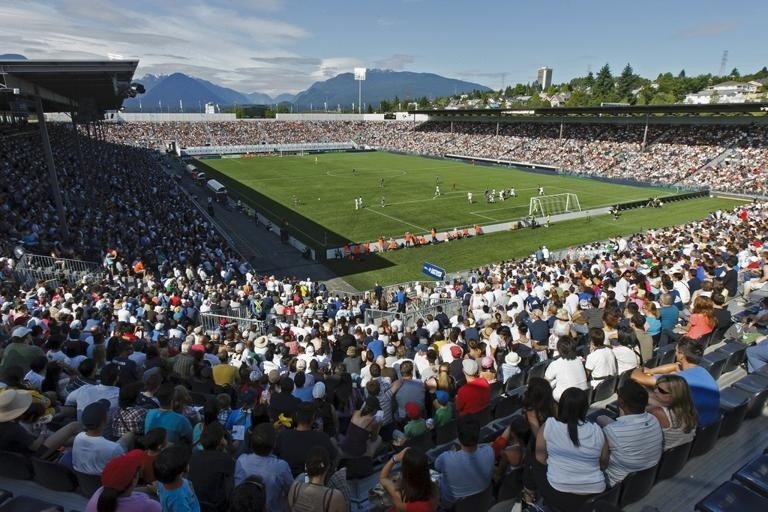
[656,383,670,394]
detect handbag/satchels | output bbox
[369,470,402,509]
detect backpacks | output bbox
[228,455,279,512]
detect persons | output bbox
[0,119,768,512]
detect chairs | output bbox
[1,342,768,512]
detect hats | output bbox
[296,359,305,370]
[12,327,32,337]
[580,299,589,310]
[0,389,32,423]
[289,341,299,356]
[534,309,546,320]
[405,402,420,417]
[436,390,450,404]
[154,443,191,482]
[254,336,268,347]
[462,359,478,375]
[450,346,462,356]
[347,346,355,356]
[414,344,428,351]
[387,344,395,353]
[83,399,110,426]
[312,382,325,398]
[505,351,521,366]
[155,323,164,331]
[556,309,570,321]
[235,343,244,353]
[103,449,144,489]
[481,357,493,368]
[269,370,280,384]
